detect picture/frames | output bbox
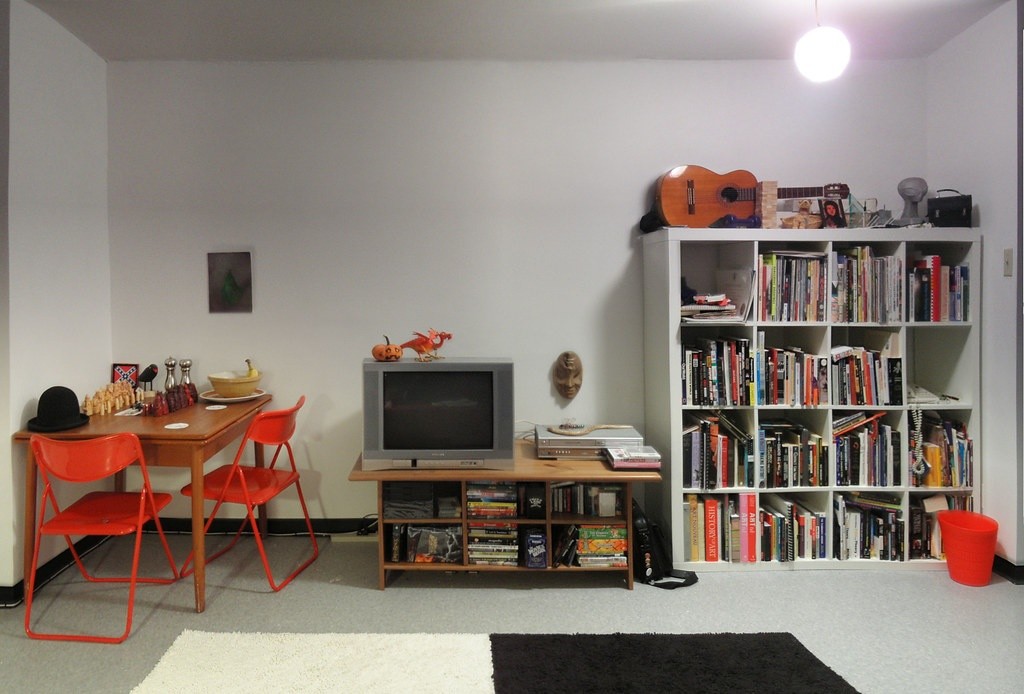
[818,197,846,229]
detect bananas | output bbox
[244,359,258,378]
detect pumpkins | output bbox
[371,336,403,361]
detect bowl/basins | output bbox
[207,371,263,398]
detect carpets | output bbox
[490,632,859,694]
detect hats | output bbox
[27,386,90,431]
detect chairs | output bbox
[25,433,179,642]
[180,395,319,591]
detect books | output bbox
[833,491,904,562]
[468,480,518,566]
[906,250,969,322]
[909,417,973,487]
[683,410,754,490]
[758,249,828,322]
[833,244,902,323]
[831,330,903,405]
[757,330,828,407]
[683,493,756,563]
[603,444,661,472]
[681,333,755,407]
[759,493,826,562]
[832,410,901,486]
[681,293,745,324]
[759,418,828,489]
[909,493,976,560]
[551,480,628,568]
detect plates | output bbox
[199,387,266,403]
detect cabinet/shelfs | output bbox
[642,229,982,572]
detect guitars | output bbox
[654,164,851,227]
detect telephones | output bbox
[907,382,939,404]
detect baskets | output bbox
[208,375,262,398]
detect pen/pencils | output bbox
[863,201,866,228]
[941,394,959,401]
[544,463,575,470]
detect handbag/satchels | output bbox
[927,189,972,227]
[633,498,698,589]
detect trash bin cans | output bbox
[938,510,998,587]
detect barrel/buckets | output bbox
[936,510,998,587]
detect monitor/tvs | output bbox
[362,358,515,470]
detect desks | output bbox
[13,393,273,613]
[347,436,661,590]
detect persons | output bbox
[824,201,845,228]
